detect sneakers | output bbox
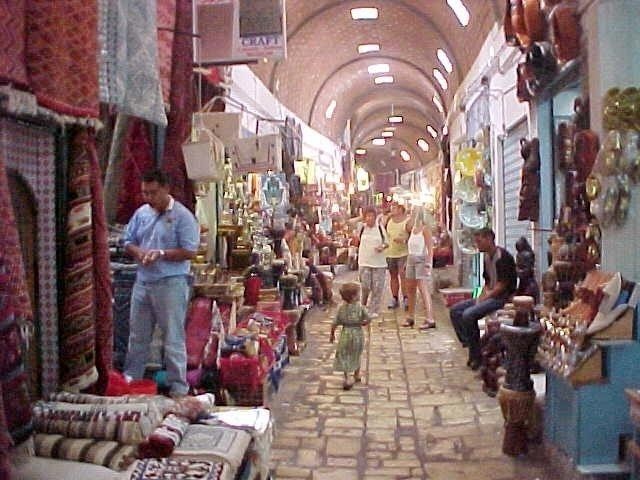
[344,374,362,389]
[467,348,482,369]
[389,297,435,329]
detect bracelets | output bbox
[159,249,166,260]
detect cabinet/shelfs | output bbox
[543,289,640,465]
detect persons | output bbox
[280,221,339,312]
[330,283,373,391]
[515,236,542,310]
[355,206,390,317]
[401,204,437,330]
[122,167,203,403]
[448,227,517,369]
[384,200,413,308]
